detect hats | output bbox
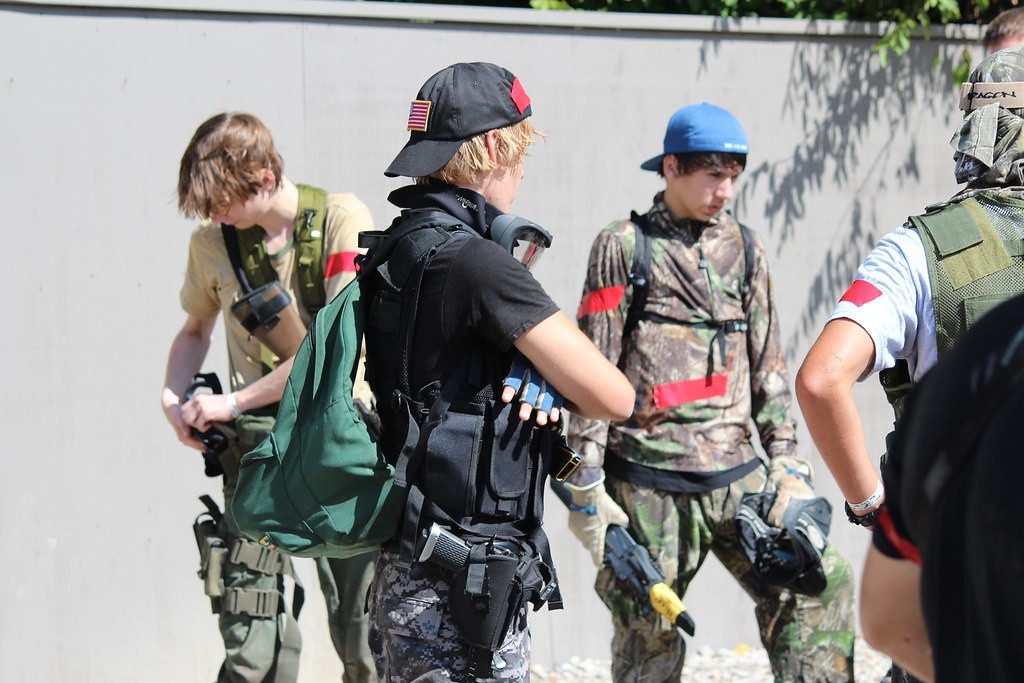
[958,45,1024,118]
[383,63,532,177]
[640,101,749,172]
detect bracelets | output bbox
[846,480,885,511]
[844,499,888,527]
[226,391,241,420]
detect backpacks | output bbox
[230,208,482,560]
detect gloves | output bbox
[764,455,817,528]
[563,469,630,571]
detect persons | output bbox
[550,100,856,683]
[159,111,378,683]
[357,62,637,683]
[794,6,1024,683]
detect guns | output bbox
[230,282,308,361]
[413,522,522,680]
[550,473,696,637]
